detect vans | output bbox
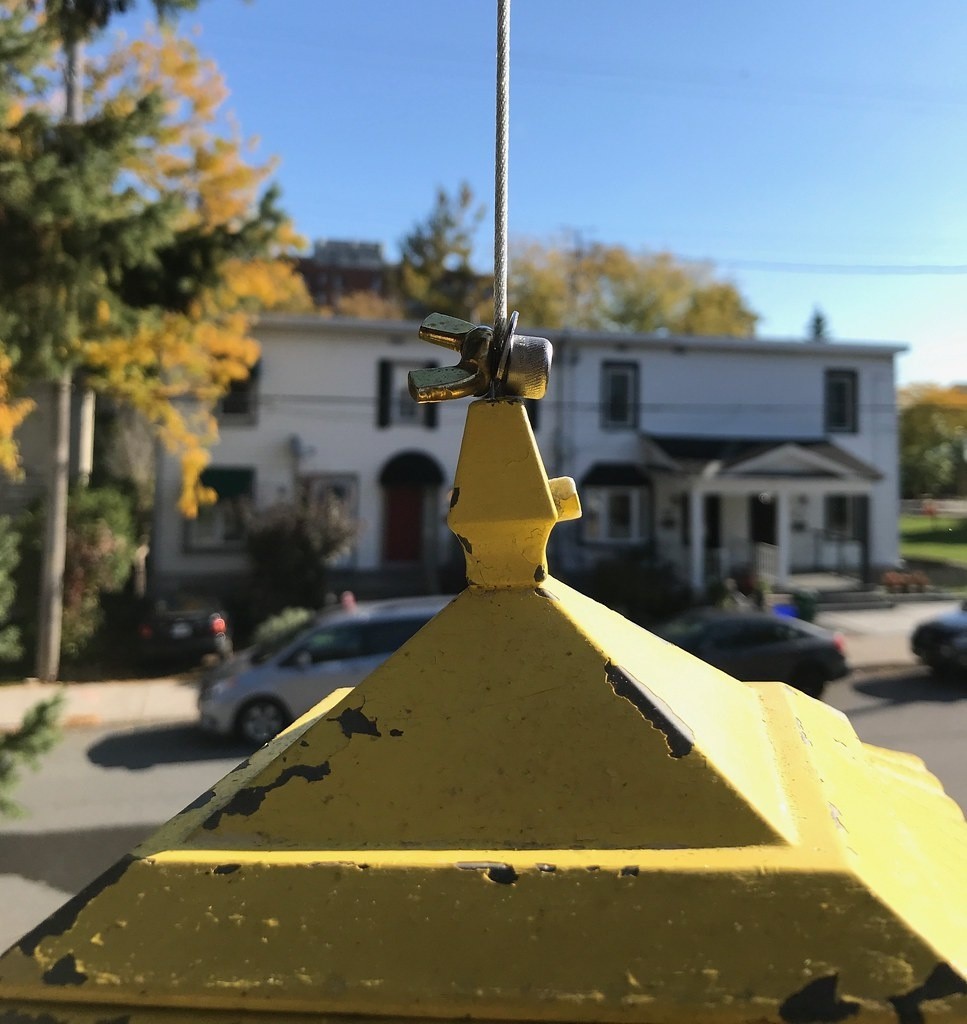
[195,594,457,750]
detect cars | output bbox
[651,613,852,703]
[136,594,233,677]
[912,599,967,680]
